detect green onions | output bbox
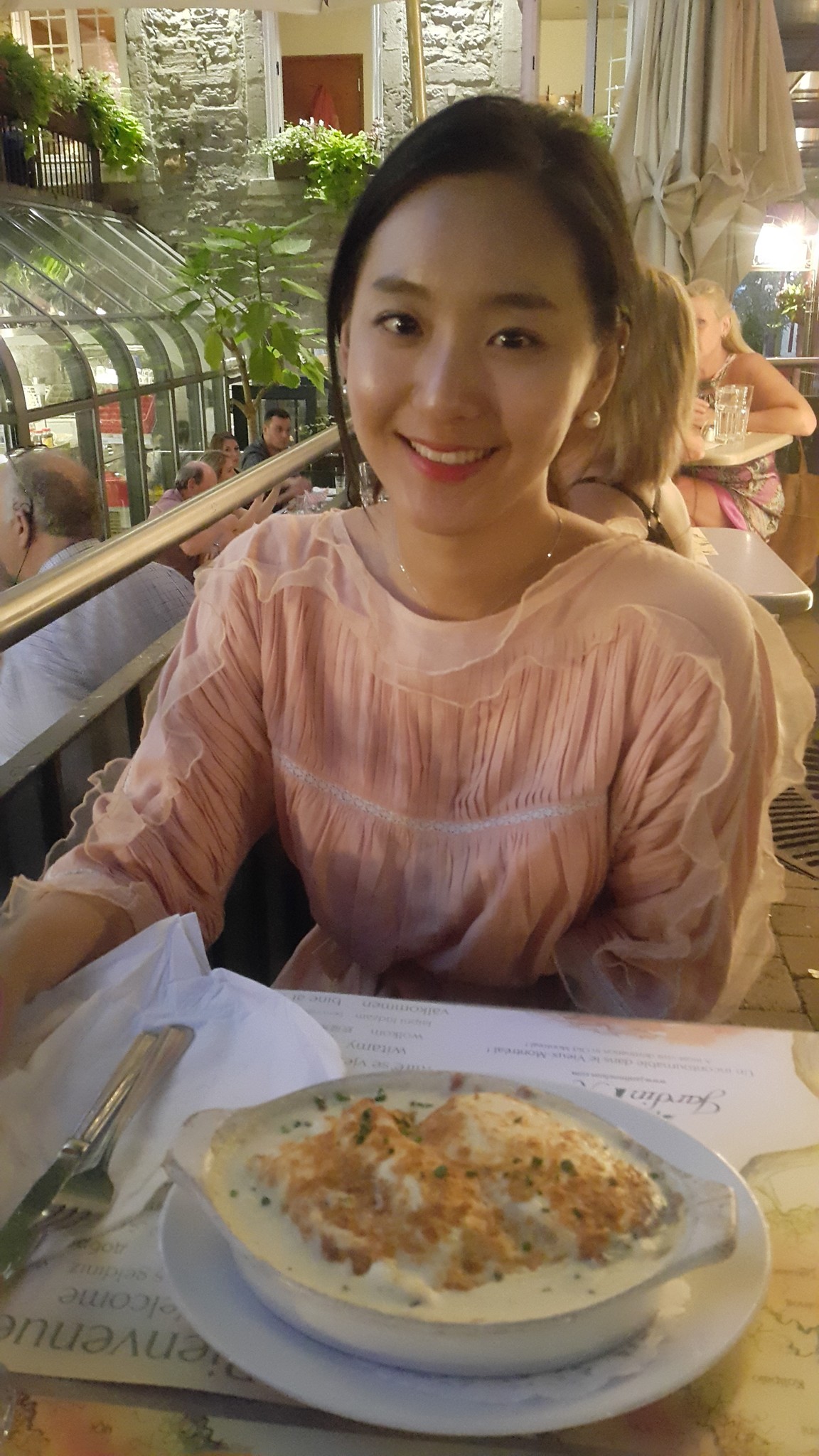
[228,1088,662,1303]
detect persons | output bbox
[193,453,281,549]
[673,279,815,540]
[150,460,235,562]
[0,453,200,844]
[0,95,815,1024]
[547,265,694,553]
[237,408,313,514]
[210,435,242,480]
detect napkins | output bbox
[0,912,348,1261]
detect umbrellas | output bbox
[606,0,805,292]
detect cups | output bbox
[713,383,754,444]
[335,467,351,508]
[358,462,389,508]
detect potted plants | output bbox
[265,123,315,179]
[47,58,91,142]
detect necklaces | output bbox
[386,505,564,618]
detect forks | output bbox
[25,1024,196,1235]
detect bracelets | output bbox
[212,542,220,547]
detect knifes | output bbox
[1,1026,160,1289]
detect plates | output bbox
[156,1079,770,1436]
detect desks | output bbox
[695,526,813,615]
[193,487,338,579]
[68,443,125,463]
[683,431,794,467]
[0,989,819,1456]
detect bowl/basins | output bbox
[163,1068,740,1378]
